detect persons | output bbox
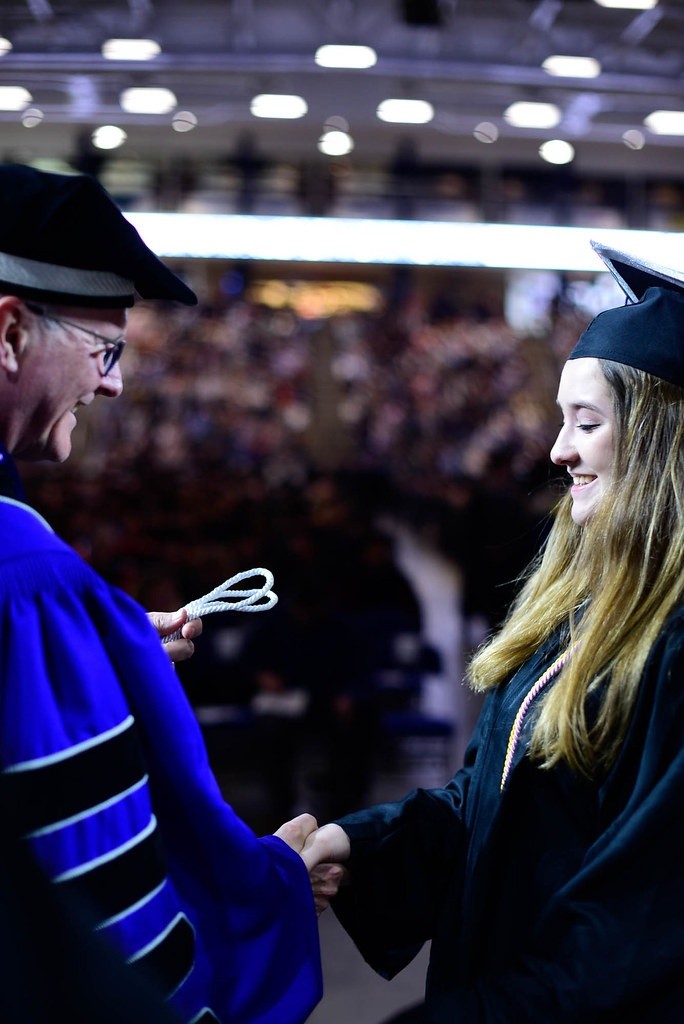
[0,221,338,1022]
[299,307,682,1023]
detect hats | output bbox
[0,159,199,308]
[568,239,683,388]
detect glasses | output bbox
[23,302,127,377]
